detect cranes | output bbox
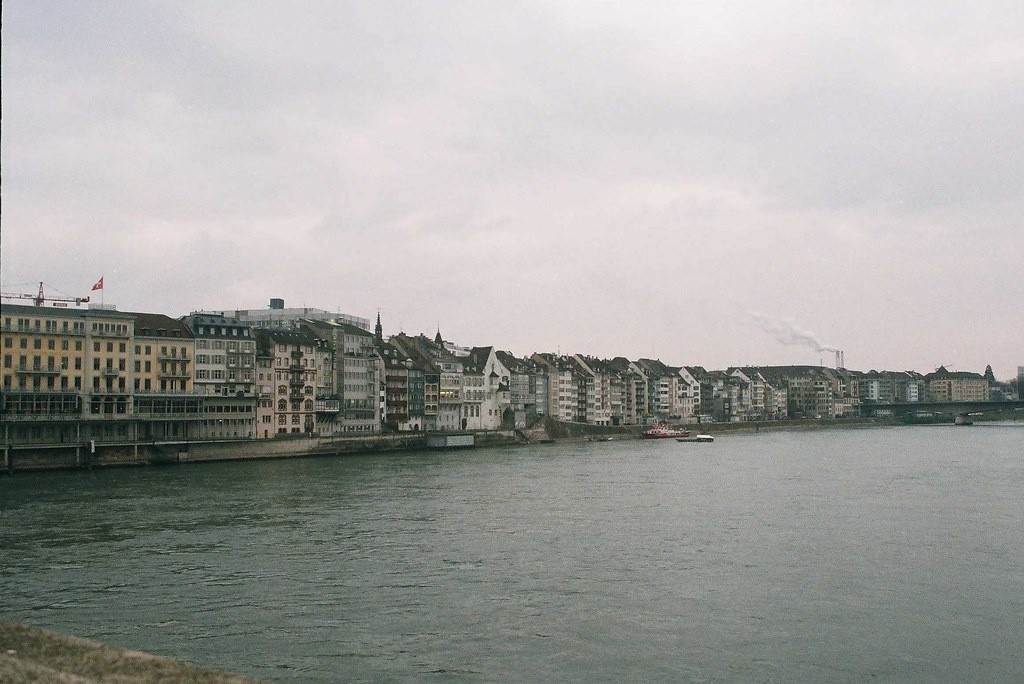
[1,281,90,307]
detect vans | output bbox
[815,414,821,418]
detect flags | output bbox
[91,277,104,290]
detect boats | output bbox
[539,439,555,443]
[642,422,690,439]
